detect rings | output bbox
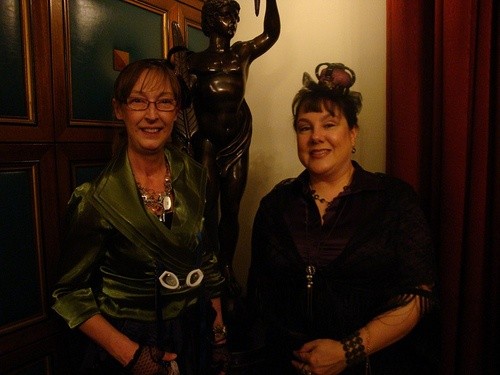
[306,369,312,375]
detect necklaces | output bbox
[311,185,350,221]
[132,162,172,221]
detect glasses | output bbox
[117,94,180,111]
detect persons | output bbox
[242,60,438,375]
[165,0,282,300]
[50,59,228,374]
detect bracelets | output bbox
[340,330,367,371]
